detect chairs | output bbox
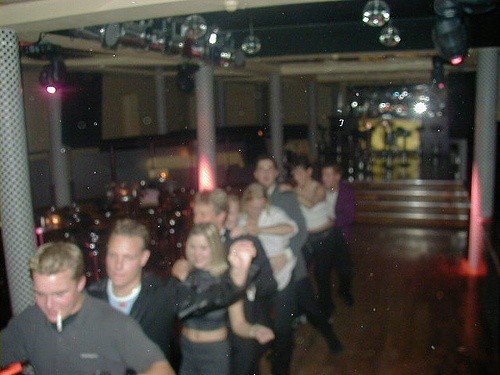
[36,173,254,283]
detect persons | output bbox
[171,153,356,375]
[83,217,189,375]
[0,241,178,375]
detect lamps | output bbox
[428,0,473,88]
[365,4,399,45]
[124,17,259,69]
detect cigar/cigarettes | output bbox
[55,309,62,333]
